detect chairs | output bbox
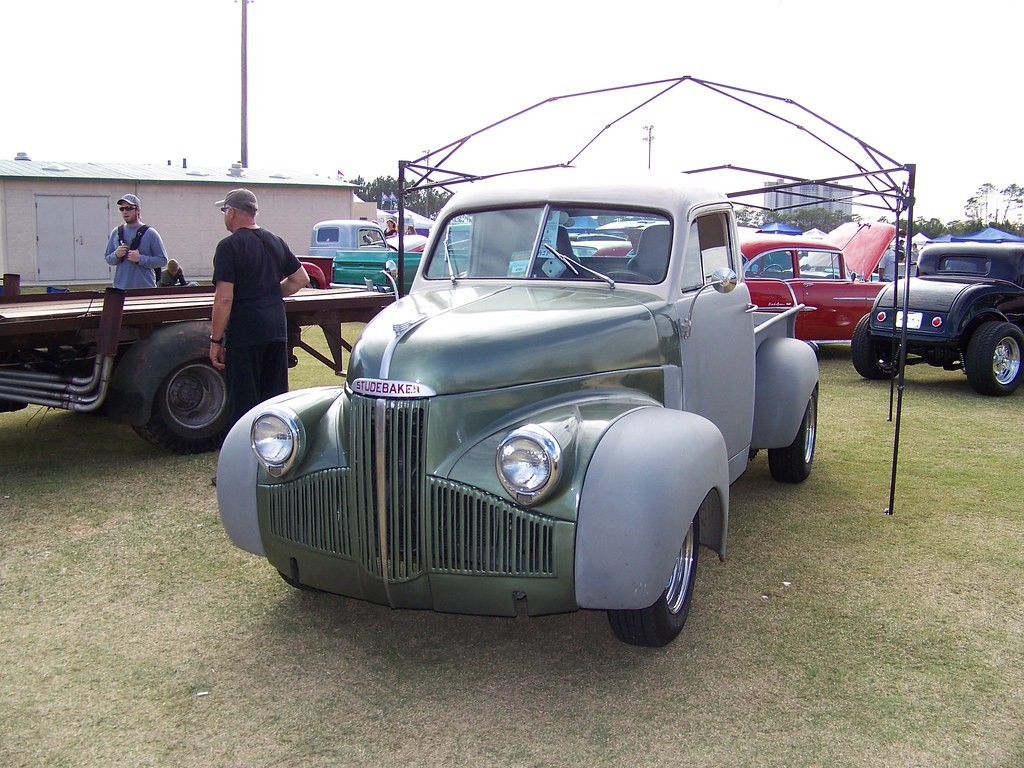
[525,222,583,273]
[628,222,673,283]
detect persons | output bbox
[104,193,168,288]
[157,259,199,287]
[878,245,894,282]
[209,188,310,485]
[405,226,417,235]
[899,240,906,259]
[384,219,398,239]
[912,243,918,259]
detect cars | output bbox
[740,221,906,340]
[851,242,1024,397]
[217,167,819,645]
[280,220,427,289]
[571,221,670,256]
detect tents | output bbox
[393,208,434,230]
[912,231,931,245]
[926,227,1024,243]
[796,228,828,239]
[755,222,802,235]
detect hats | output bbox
[116,194,140,208]
[214,188,258,211]
[168,259,179,272]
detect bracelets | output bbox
[210,335,223,344]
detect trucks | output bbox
[329,223,470,294]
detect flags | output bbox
[338,170,344,176]
[392,194,397,203]
[383,194,390,202]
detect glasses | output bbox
[119,207,137,211]
[221,207,229,214]
